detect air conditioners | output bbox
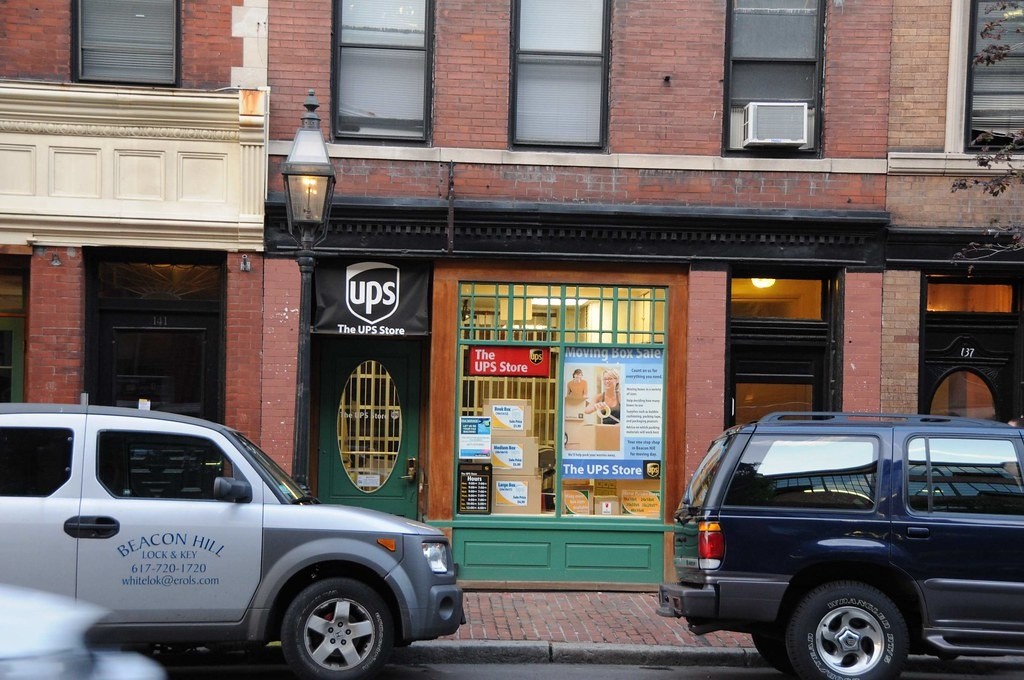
[742,102,809,149]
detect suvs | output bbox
[0,399,465,680]
[652,413,1024,679]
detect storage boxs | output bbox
[481,398,661,515]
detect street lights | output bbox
[282,88,338,504]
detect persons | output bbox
[584,368,621,424]
[567,369,588,399]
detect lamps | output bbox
[750,278,776,289]
